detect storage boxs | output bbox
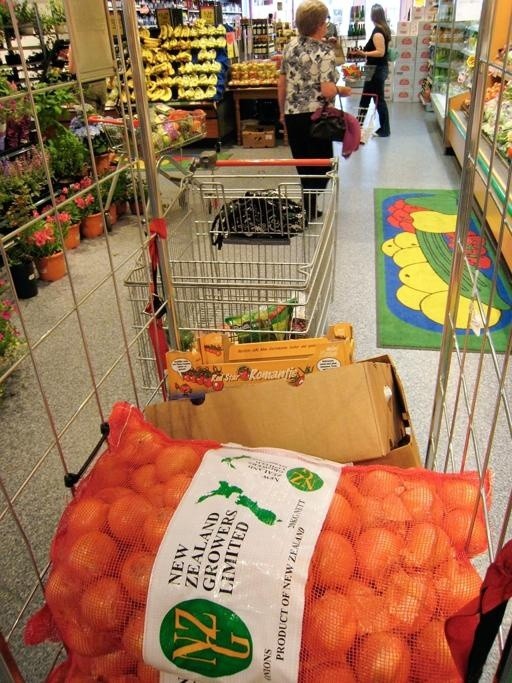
[384,5,432,101]
[144,350,424,471]
[241,124,275,147]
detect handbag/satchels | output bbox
[208,188,308,250]
[310,84,348,142]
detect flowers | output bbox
[24,174,99,254]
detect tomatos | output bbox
[179,368,225,395]
[238,366,250,380]
[388,199,492,269]
[287,370,300,380]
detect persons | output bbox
[278,0,352,224]
[355,3,391,138]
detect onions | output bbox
[45,430,200,683]
[299,466,488,683]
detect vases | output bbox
[35,252,68,281]
[82,213,105,238]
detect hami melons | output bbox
[381,210,501,330]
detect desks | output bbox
[224,86,289,146]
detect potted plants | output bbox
[98,167,149,228]
[7,247,38,298]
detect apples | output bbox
[231,63,277,85]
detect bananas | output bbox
[105,18,227,103]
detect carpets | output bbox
[372,186,512,353]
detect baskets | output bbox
[341,64,377,81]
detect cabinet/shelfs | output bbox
[0,0,147,292]
[425,0,512,201]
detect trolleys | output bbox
[122,149,341,402]
